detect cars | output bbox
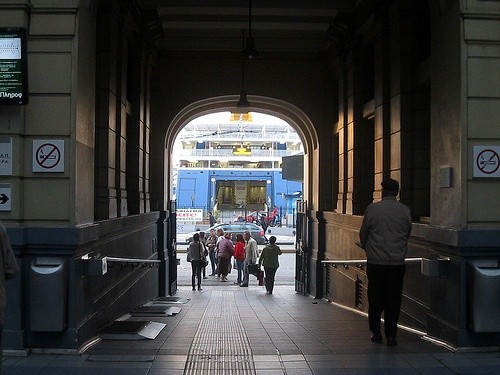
[236,211,278,225]
[186,223,265,246]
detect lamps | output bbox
[235,39,250,113]
[241,0,260,61]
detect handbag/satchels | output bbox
[201,252,208,267]
[204,250,208,256]
[259,271,264,286]
[194,260,201,266]
[187,254,192,262]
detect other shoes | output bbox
[234,282,241,285]
[209,271,229,283]
[196,276,208,279]
[239,283,248,287]
[371,335,382,342]
[267,289,272,294]
[387,339,397,346]
[198,289,202,291]
[192,289,196,291]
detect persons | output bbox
[187,212,282,294]
[354,179,411,346]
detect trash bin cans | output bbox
[28,257,67,332]
[468,260,500,333]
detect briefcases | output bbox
[247,263,259,274]
